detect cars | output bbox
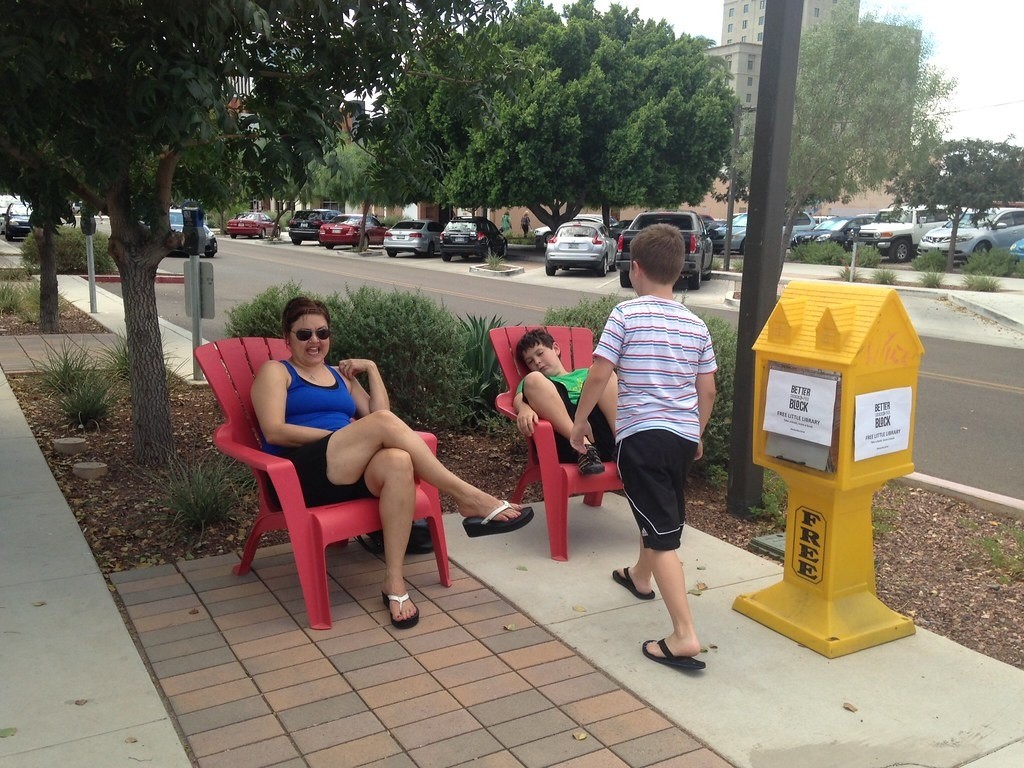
[533,209,726,255]
[917,207,1024,267]
[317,213,391,250]
[543,219,619,277]
[789,213,877,256]
[0,200,34,241]
[148,208,218,258]
[709,210,816,256]
[226,211,281,239]
[382,219,446,258]
[71,201,83,215]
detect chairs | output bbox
[194,337,452,630]
[489,326,623,562]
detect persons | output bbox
[501,211,513,232]
[512,328,620,474]
[569,222,718,669]
[520,211,532,238]
[250,296,536,629]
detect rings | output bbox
[344,362,346,366]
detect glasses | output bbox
[289,327,331,341]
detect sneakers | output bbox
[578,444,605,477]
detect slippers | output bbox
[642,640,706,669]
[613,566,655,600]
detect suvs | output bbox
[438,215,508,262]
[844,204,966,264]
[288,208,344,246]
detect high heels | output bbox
[462,499,534,537]
[383,593,419,629]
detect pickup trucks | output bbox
[614,210,715,291]
